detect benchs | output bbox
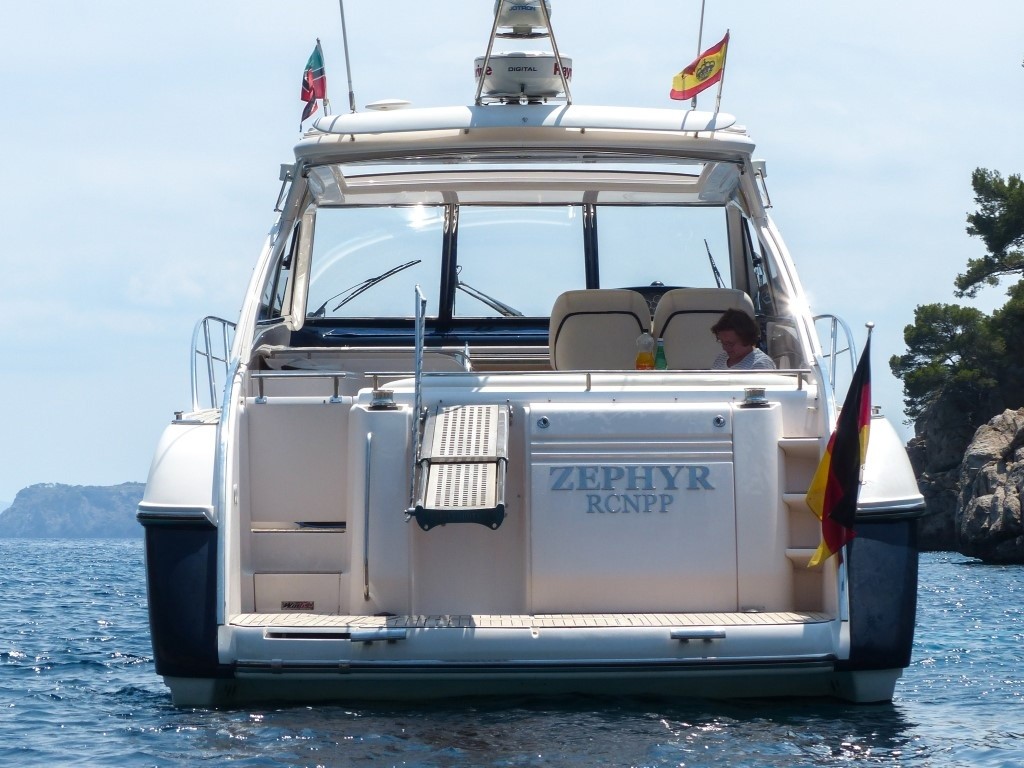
[290,323,550,359]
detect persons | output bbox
[709,309,777,369]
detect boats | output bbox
[133,1,929,705]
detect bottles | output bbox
[654,338,668,370]
[635,328,654,369]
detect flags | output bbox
[670,33,727,98]
[299,46,324,130]
[807,338,872,564]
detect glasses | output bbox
[716,338,744,347]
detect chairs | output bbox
[655,288,756,370]
[550,288,651,370]
